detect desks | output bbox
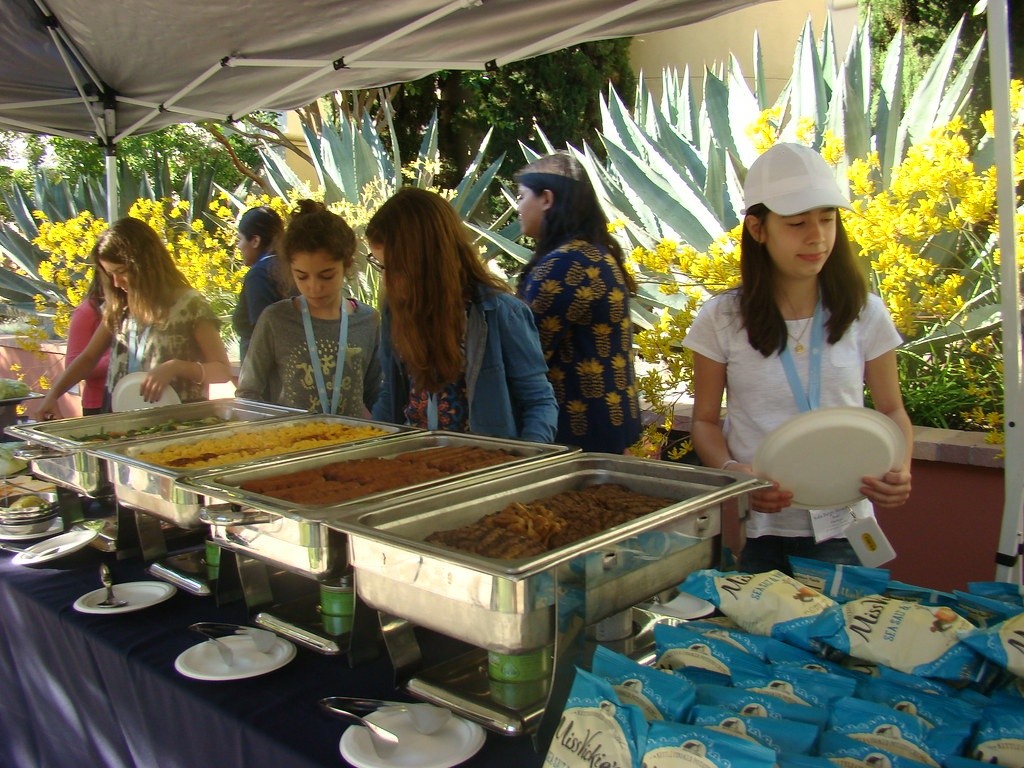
[0,392,46,442]
[0,469,726,768]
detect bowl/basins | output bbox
[0,476,59,534]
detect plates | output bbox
[71,581,177,613]
[338,705,485,768]
[752,408,906,510]
[173,635,297,681]
[0,517,99,566]
[110,373,182,413]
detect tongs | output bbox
[188,620,276,668]
[317,696,442,747]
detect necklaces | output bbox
[788,307,813,353]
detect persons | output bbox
[232,207,296,366]
[35,217,231,423]
[514,154,643,456]
[363,186,558,444]
[682,143,914,580]
[235,200,381,420]
[66,269,112,416]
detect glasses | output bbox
[366,253,387,275]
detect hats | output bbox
[744,142,854,217]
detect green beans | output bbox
[69,415,223,441]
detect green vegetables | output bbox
[0,377,31,400]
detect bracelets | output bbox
[721,460,738,470]
[192,362,205,385]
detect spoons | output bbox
[97,563,127,607]
